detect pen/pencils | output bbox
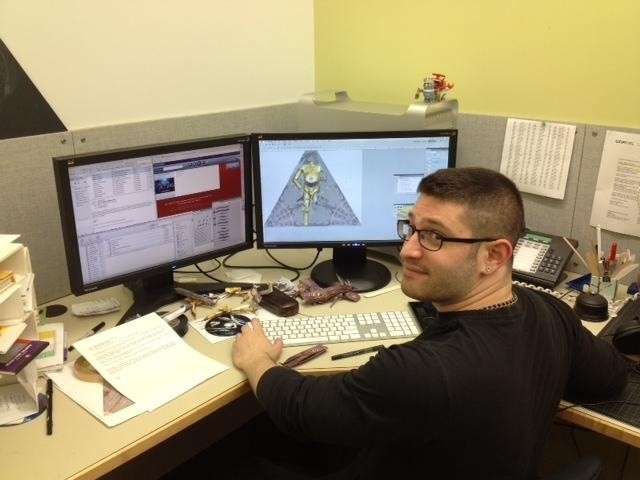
[68,321,107,350]
[332,345,384,360]
[561,224,630,277]
[174,287,212,305]
[46,379,53,435]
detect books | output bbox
[0,268,67,386]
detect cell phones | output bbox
[257,285,301,318]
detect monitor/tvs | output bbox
[251,131,458,293]
[53,134,265,323]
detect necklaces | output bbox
[478,289,515,312]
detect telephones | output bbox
[511,229,579,288]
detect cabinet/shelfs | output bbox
[0,243,39,387]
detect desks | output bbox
[0,233,640,480]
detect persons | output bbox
[230,165,629,480]
[293,155,326,226]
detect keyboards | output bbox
[245,310,419,348]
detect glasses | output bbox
[397,220,498,252]
